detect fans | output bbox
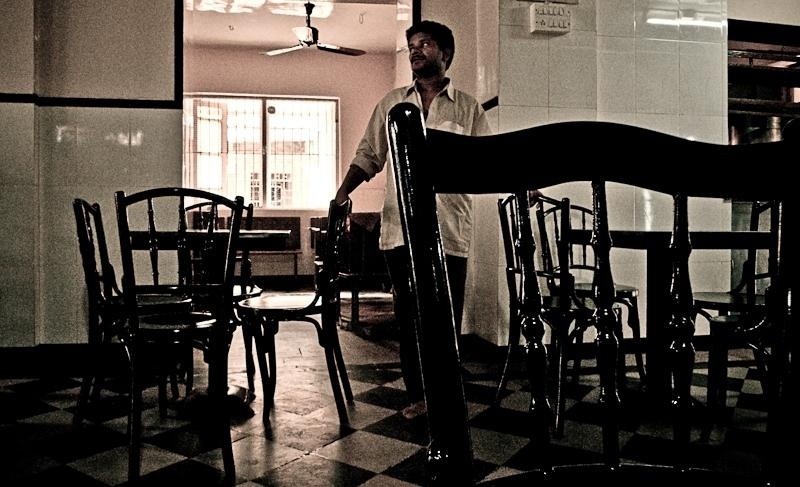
[266,0,366,57]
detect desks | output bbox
[562,223,771,457]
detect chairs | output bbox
[385,101,798,487]
[185,197,396,331]
[73,197,239,484]
[491,191,624,431]
[70,101,799,486]
[109,187,244,426]
[537,202,650,404]
[238,195,353,435]
[689,201,785,415]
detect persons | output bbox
[335,20,497,421]
